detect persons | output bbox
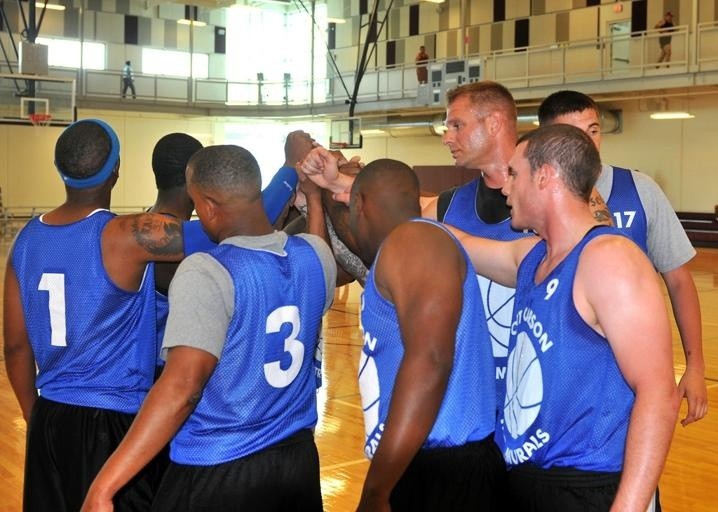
[3,118,316,510]
[538,92,711,428]
[653,12,676,70]
[146,132,294,382]
[299,82,612,415]
[322,158,506,512]
[75,145,337,510]
[414,45,428,83]
[333,122,679,512]
[122,59,136,98]
